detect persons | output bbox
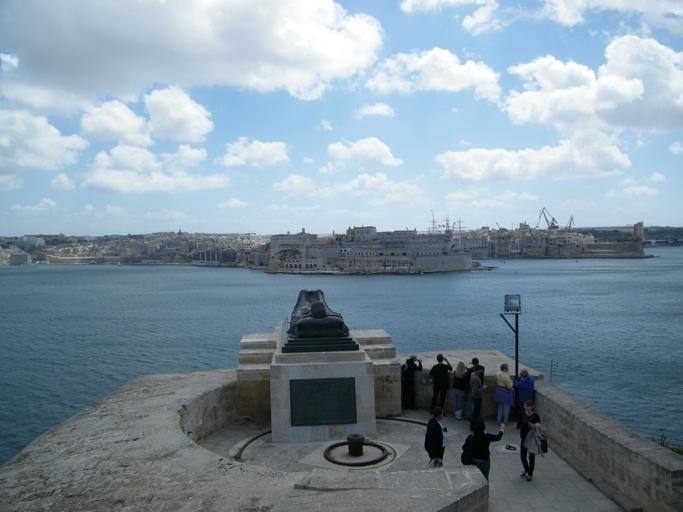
[496,363,514,428]
[400,354,422,410]
[465,357,485,416]
[512,368,536,430]
[469,364,488,419]
[428,353,453,418]
[424,406,451,468]
[452,361,469,421]
[461,417,506,483]
[512,399,542,481]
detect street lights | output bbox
[498,294,523,382]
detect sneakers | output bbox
[453,410,507,428]
[520,471,532,481]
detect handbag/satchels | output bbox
[461,450,473,464]
[538,439,547,454]
